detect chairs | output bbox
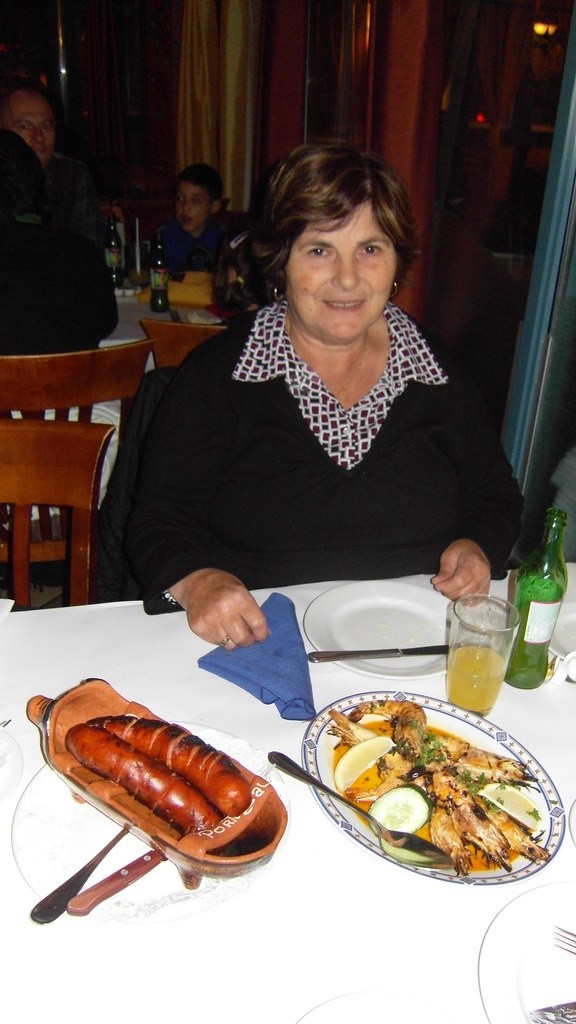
[0,318,234,607]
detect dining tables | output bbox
[77,290,228,510]
[3,566,576,1023]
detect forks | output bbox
[553,925,576,955]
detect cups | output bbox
[446,594,519,718]
[123,239,151,287]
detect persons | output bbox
[126,140,525,652]
[147,162,239,274]
[0,73,120,592]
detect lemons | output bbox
[477,783,541,830]
[335,736,395,792]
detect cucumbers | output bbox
[368,784,432,836]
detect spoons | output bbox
[266,752,456,869]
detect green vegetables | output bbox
[390,721,541,820]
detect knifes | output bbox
[530,1002,576,1024]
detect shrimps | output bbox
[349,700,427,758]
[330,707,549,874]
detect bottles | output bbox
[507,508,568,691]
[103,211,121,289]
[149,233,170,312]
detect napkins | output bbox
[197,592,320,720]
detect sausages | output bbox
[66,716,251,834]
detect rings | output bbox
[219,636,230,647]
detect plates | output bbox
[478,883,576,1024]
[302,690,565,887]
[295,991,383,1024]
[548,600,576,660]
[305,583,461,680]
[28,677,288,887]
[1,718,289,923]
